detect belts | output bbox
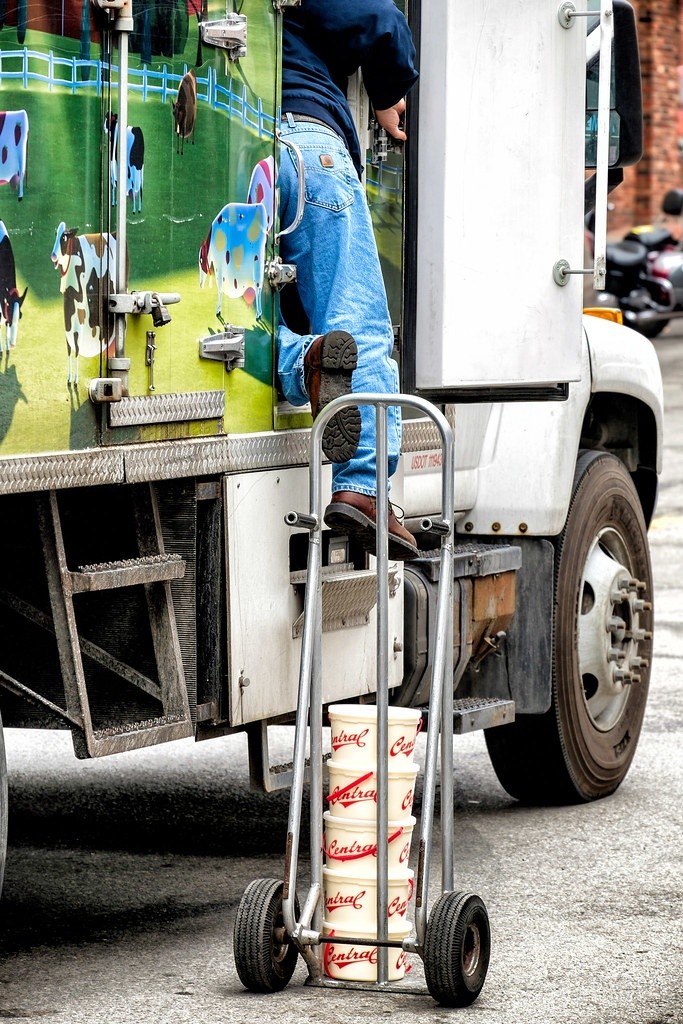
[282,113,339,135]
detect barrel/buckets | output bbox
[321,704,422,983]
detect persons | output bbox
[279,0,418,560]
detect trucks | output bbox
[0,0,667,852]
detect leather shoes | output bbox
[304,330,362,464]
[324,492,420,561]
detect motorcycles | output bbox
[599,189,683,341]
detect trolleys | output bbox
[232,388,495,1009]
